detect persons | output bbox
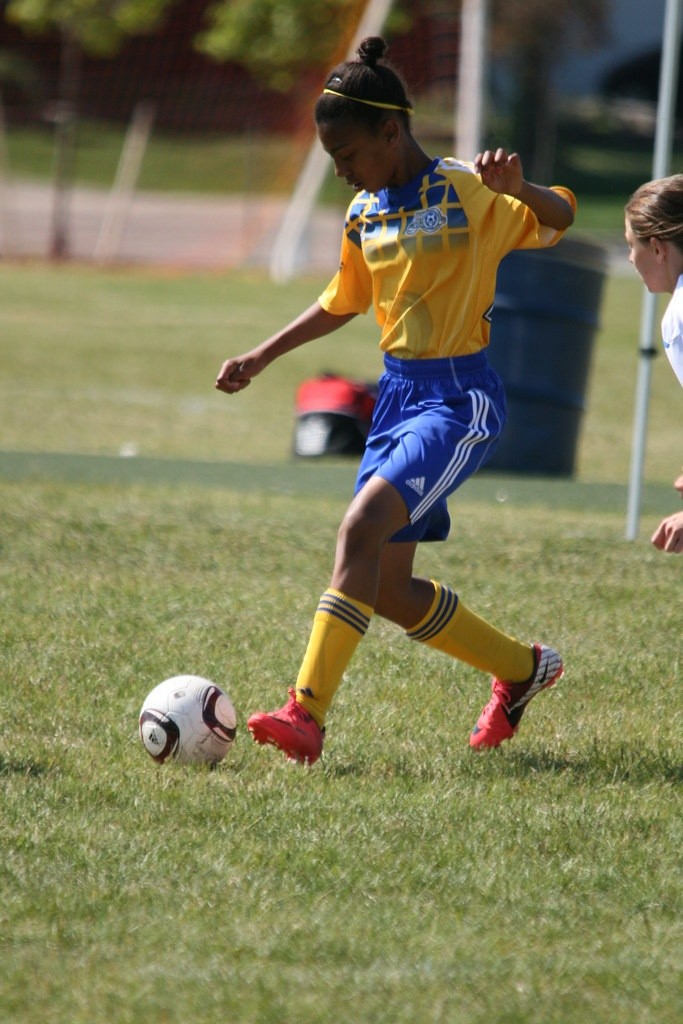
[620,172,683,556]
[212,35,582,771]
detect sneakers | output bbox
[247,689,326,765]
[468,644,564,751]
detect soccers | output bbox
[134,671,244,774]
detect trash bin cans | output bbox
[474,234,613,481]
